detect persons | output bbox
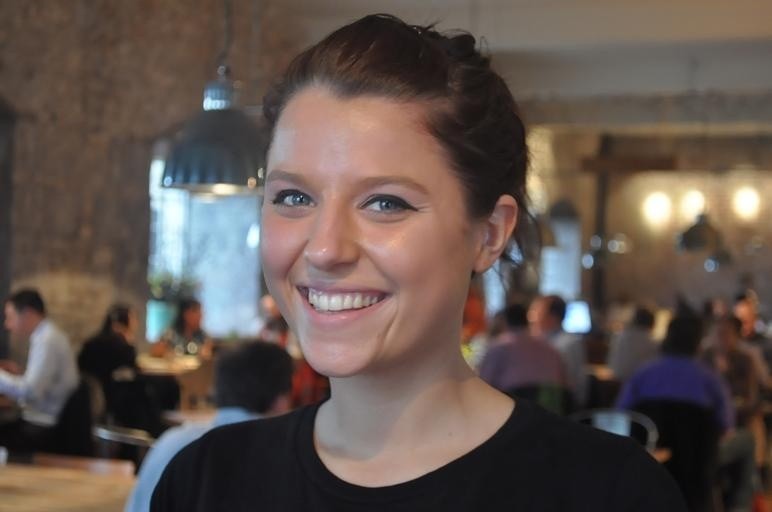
[0,284,84,459]
[78,300,184,441]
[260,295,290,349]
[458,273,771,509]
[150,298,215,364]
[122,12,682,510]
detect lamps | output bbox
[536,155,735,253]
[162,0,266,192]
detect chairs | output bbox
[463,310,772,511]
[1,313,319,511]
